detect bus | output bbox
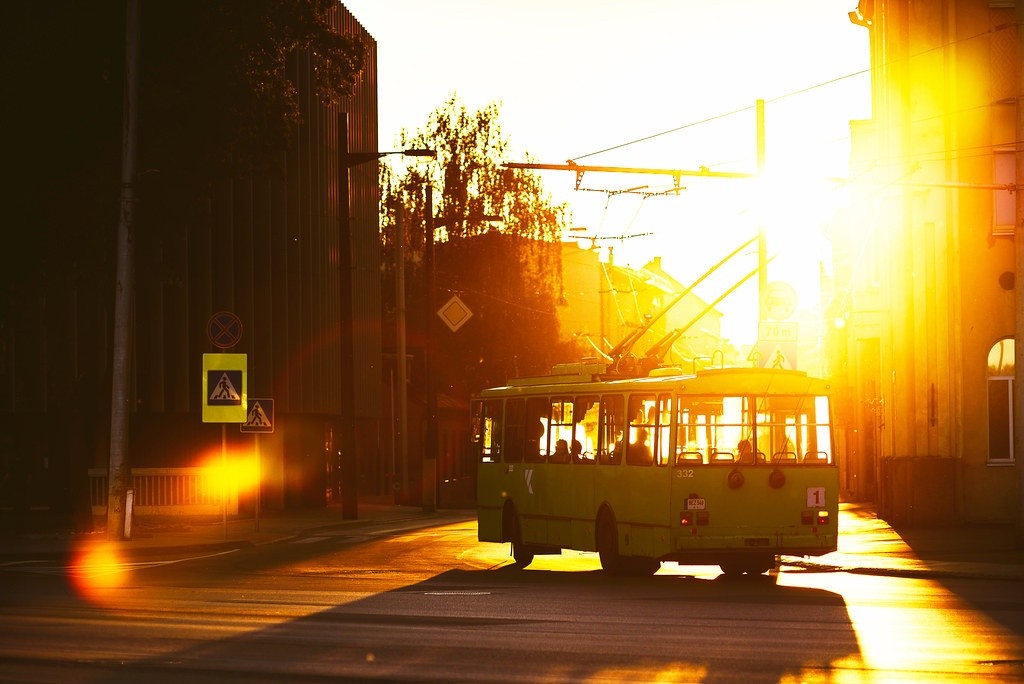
[469,227,840,582]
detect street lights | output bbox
[336,107,437,523]
[424,184,505,513]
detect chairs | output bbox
[677,452,828,464]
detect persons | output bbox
[551,429,763,464]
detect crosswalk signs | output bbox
[202,352,248,424]
[239,397,274,434]
